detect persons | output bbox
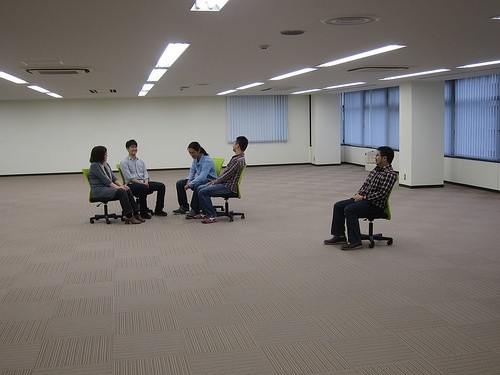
[89,146,145,224]
[324,145,398,249]
[119,139,167,219]
[194,136,248,222]
[173,141,217,219]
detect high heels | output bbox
[125,216,141,224]
[134,214,145,222]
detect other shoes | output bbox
[194,213,207,219]
[140,213,151,219]
[173,207,186,214]
[155,211,167,216]
[186,209,200,219]
[201,216,217,223]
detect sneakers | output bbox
[325,236,347,245]
[342,240,363,250]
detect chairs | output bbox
[82,169,125,224]
[199,158,248,222]
[116,164,155,217]
[360,173,399,248]
